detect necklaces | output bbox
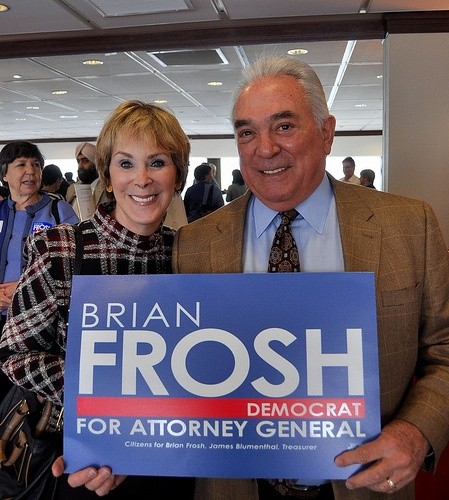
[15,191,37,208]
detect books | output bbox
[73,183,96,223]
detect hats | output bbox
[74,143,96,165]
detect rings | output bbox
[387,479,395,490]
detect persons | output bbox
[209,162,217,178]
[0,98,192,500]
[40,164,81,202]
[359,169,377,191]
[51,47,449,500]
[184,164,225,224]
[0,185,10,201]
[337,156,361,186]
[66,142,115,222]
[193,162,220,189]
[226,169,247,202]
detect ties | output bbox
[267,210,301,496]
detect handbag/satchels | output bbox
[199,185,216,217]
[0,392,64,500]
